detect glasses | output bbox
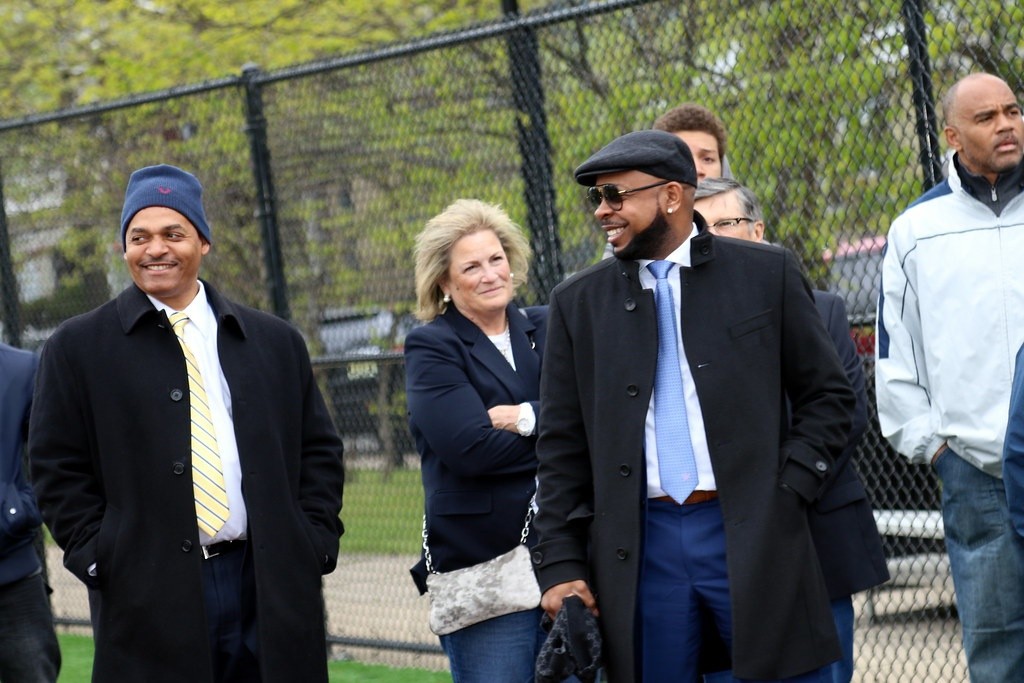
[588,178,672,211]
[705,217,754,233]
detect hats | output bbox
[575,130,697,186]
[120,163,211,242]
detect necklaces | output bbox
[495,323,511,356]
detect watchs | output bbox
[514,402,534,437]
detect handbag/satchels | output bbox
[426,544,542,637]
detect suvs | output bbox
[319,303,429,451]
[804,237,946,556]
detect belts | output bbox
[654,490,719,505]
[202,538,247,563]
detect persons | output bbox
[694,179,893,683]
[652,108,724,176]
[403,200,543,681]
[0,328,61,683]
[873,70,1023,683]
[526,129,893,682]
[26,162,347,683]
